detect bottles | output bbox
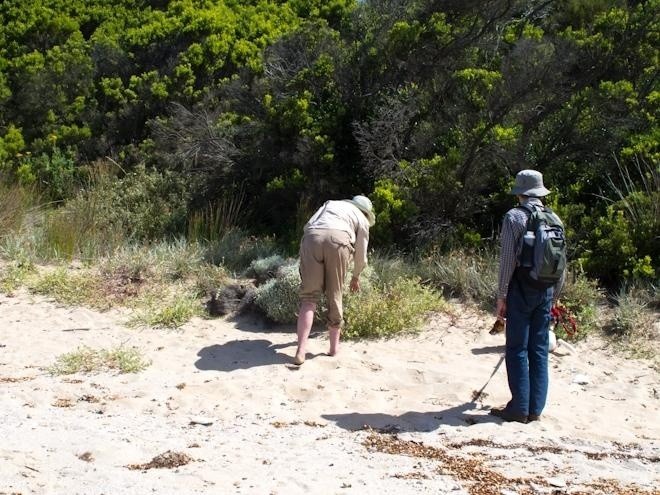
[521,230,536,269]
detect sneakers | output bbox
[529,413,541,423]
[491,406,528,424]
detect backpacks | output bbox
[516,203,568,286]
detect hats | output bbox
[510,169,552,198]
[343,195,376,228]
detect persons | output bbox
[490,167,568,425]
[294,194,378,368]
